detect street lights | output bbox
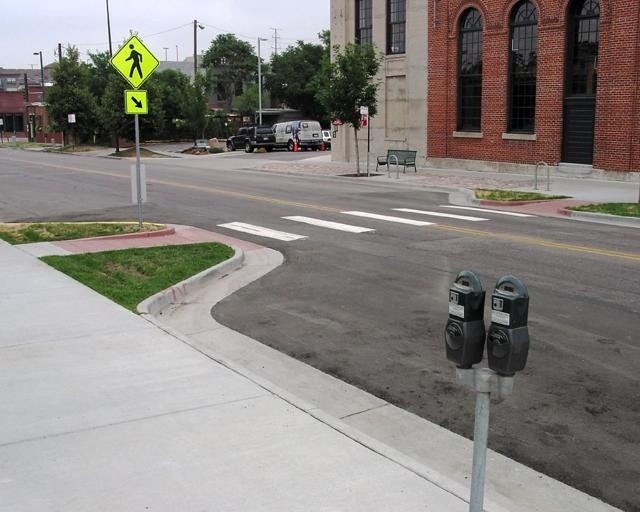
[258,38,267,124]
[34,50,44,102]
[193,20,205,78]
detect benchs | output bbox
[376,149,418,174]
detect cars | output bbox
[322,130,331,149]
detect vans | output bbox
[272,120,323,150]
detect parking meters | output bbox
[444,270,529,511]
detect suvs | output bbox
[226,125,276,153]
